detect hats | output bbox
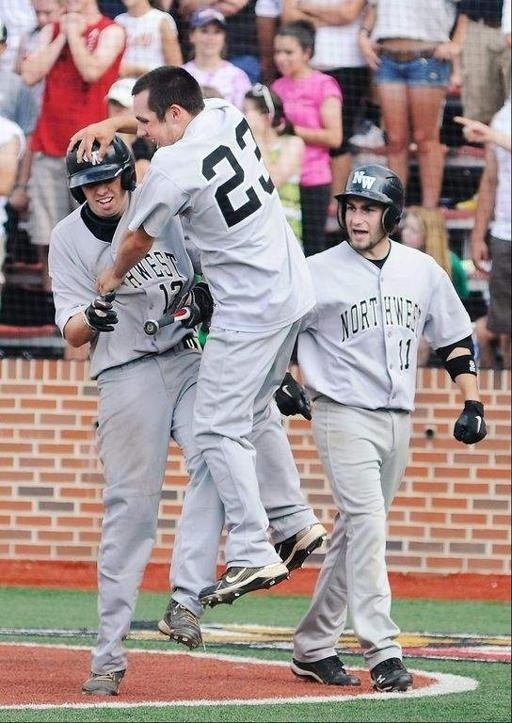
[188,8,230,32]
[102,77,140,111]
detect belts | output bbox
[378,44,437,63]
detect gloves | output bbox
[273,370,313,423]
[452,400,488,445]
[82,290,119,333]
[175,281,214,330]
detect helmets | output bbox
[64,132,137,205]
[331,163,405,240]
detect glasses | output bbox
[249,80,277,120]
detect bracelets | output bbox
[362,26,370,36]
[13,184,28,191]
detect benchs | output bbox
[0,83,491,352]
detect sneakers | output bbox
[80,663,126,698]
[369,657,414,692]
[198,554,289,608]
[288,651,361,687]
[272,523,328,571]
[155,596,205,652]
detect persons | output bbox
[274,164,484,691]
[22,0,126,303]
[269,18,342,260]
[47,135,227,695]
[178,8,252,117]
[453,114,511,154]
[65,67,330,608]
[398,205,489,372]
[473,99,511,369]
[243,81,305,260]
[1,116,26,291]
[1,1,511,241]
[101,76,157,187]
